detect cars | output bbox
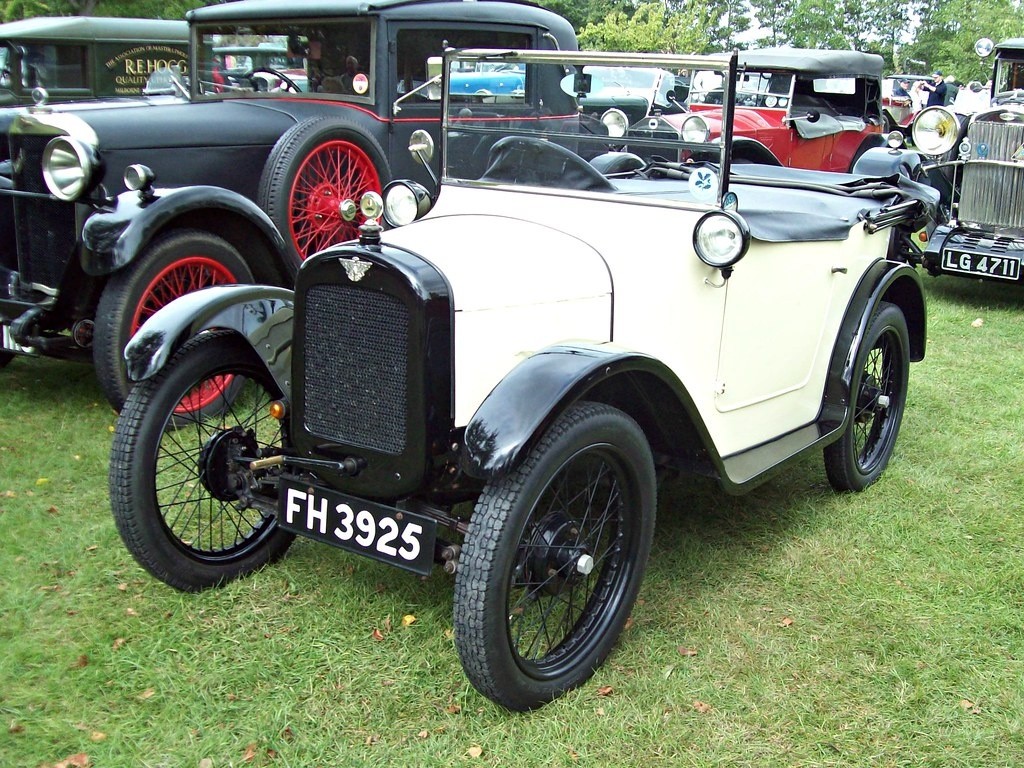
[106,45,945,714]
[1,1,1023,435]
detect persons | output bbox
[226,55,236,69]
[894,71,966,142]
[477,62,519,72]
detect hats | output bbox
[900,78,911,83]
[932,71,942,77]
